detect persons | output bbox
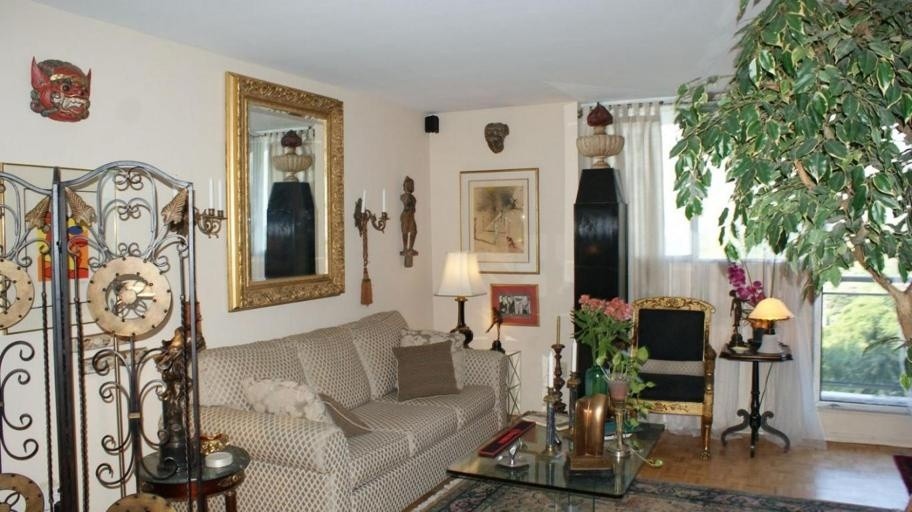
[729,290,750,334]
[499,295,530,316]
[486,307,503,341]
[400,175,416,252]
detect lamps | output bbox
[748,298,795,354]
[267,130,312,278]
[574,104,629,334]
[433,249,488,348]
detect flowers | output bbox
[724,246,766,309]
[570,295,634,366]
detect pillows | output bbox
[320,393,371,438]
[390,339,461,400]
[396,327,464,391]
[241,374,335,425]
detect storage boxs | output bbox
[521,411,570,445]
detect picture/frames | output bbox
[459,168,542,276]
[2,162,118,336]
[489,283,541,328]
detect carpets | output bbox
[412,478,906,512]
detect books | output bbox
[604,419,633,440]
[521,410,569,430]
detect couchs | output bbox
[168,312,510,512]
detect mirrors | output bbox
[226,69,344,312]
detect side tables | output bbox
[719,352,794,458]
[508,350,521,427]
[135,445,250,512]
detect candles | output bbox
[382,189,386,212]
[361,189,367,214]
[207,178,223,214]
[556,315,562,342]
[547,351,554,389]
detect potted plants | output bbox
[596,346,664,466]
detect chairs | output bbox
[631,296,716,461]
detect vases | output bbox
[585,366,607,397]
